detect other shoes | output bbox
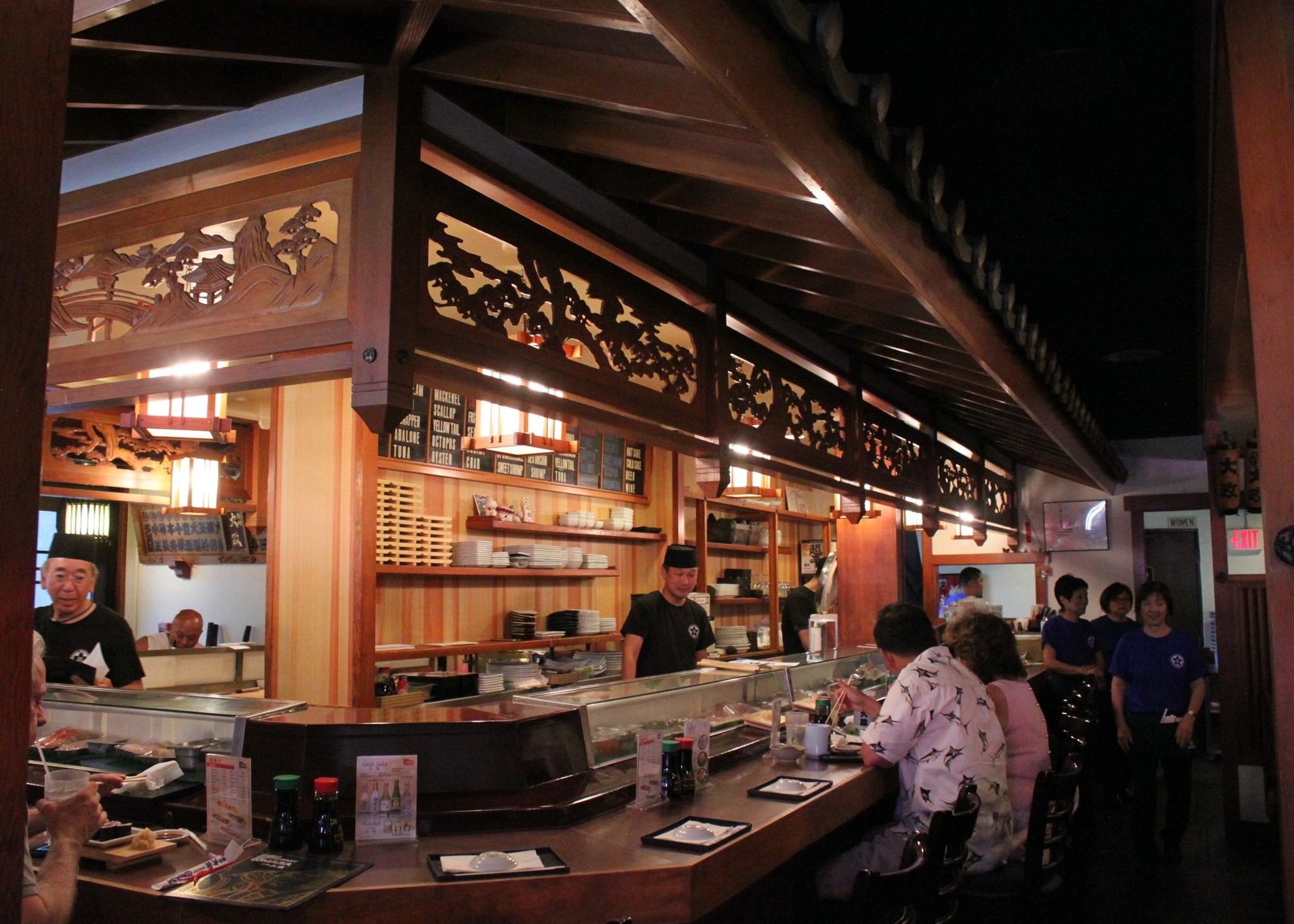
[1164,832,1182,862]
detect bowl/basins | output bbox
[767,501,782,508]
[758,530,782,548]
[769,743,805,762]
[509,609,616,639]
[562,547,583,569]
[558,507,634,531]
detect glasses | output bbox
[47,572,95,586]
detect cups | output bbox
[44,769,90,845]
[767,582,791,596]
[785,711,831,756]
[376,671,389,684]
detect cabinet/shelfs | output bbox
[682,491,792,663]
[375,515,668,674]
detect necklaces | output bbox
[50,599,93,623]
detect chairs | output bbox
[838,680,1093,924]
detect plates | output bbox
[469,852,517,872]
[561,650,623,677]
[450,540,562,568]
[673,825,716,841]
[707,584,740,600]
[153,829,189,843]
[478,660,539,694]
[579,554,608,570]
[771,779,806,791]
[715,626,751,653]
[832,722,867,738]
[88,827,145,848]
[835,744,861,753]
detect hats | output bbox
[664,544,699,568]
[47,534,96,564]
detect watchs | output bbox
[1186,710,1196,718]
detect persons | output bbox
[621,544,717,681]
[20,531,205,924]
[937,569,1206,885]
[782,557,830,653]
[814,601,1008,924]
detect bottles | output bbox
[268,775,346,853]
[814,696,833,748]
[397,677,407,694]
[1018,622,1023,634]
[762,631,770,646]
[662,738,695,805]
[750,574,769,596]
[360,779,412,835]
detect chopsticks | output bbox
[825,674,853,728]
[95,775,147,784]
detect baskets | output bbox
[546,671,579,684]
[379,692,425,708]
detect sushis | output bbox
[90,819,132,842]
[33,727,91,748]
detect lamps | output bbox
[901,496,947,537]
[830,476,882,524]
[695,359,783,499]
[119,256,237,445]
[461,270,583,457]
[954,511,987,546]
[160,441,225,517]
